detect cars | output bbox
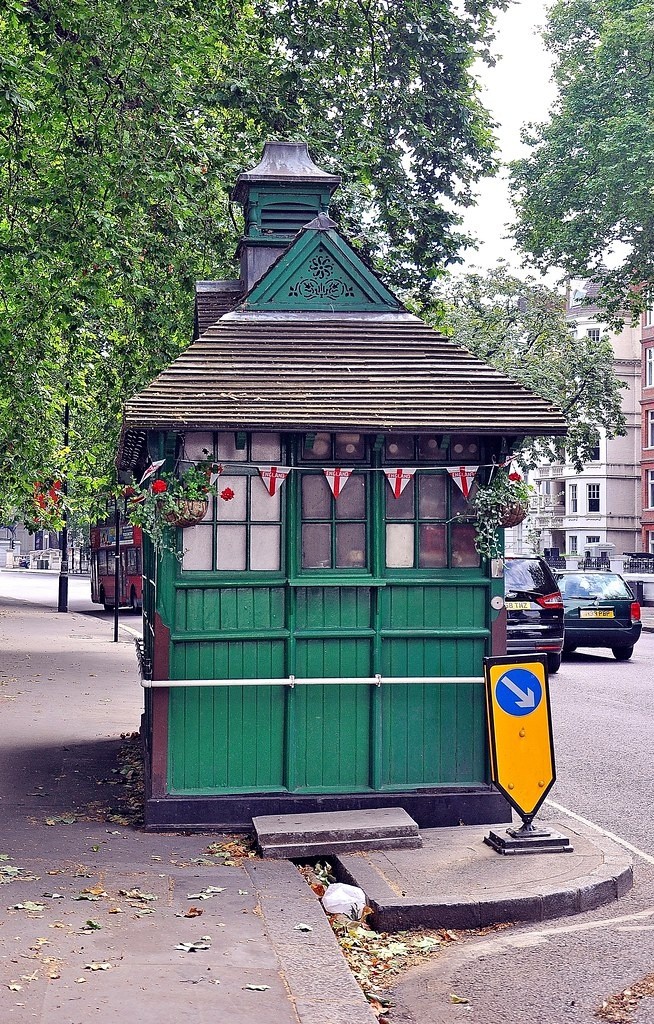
[20,557,30,568]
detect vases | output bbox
[495,500,529,528]
[157,498,209,528]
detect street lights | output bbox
[45,534,48,549]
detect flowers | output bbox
[129,453,236,564]
[471,471,544,571]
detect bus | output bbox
[86,485,142,611]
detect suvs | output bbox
[506,552,564,672]
[552,567,642,660]
